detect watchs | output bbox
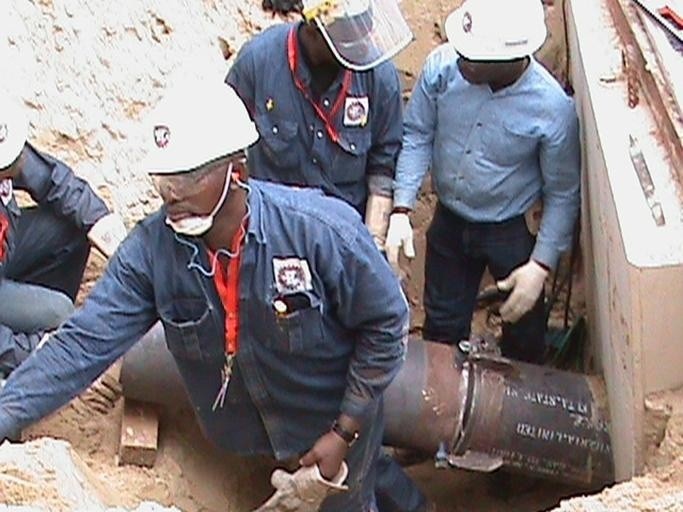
[391,207,414,216]
[330,421,361,448]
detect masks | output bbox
[165,162,235,238]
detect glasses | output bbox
[147,152,247,198]
[337,27,377,52]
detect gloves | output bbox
[366,196,416,268]
[497,261,549,325]
[79,373,123,414]
[253,459,350,512]
[86,211,127,258]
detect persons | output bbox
[0,75,437,512]
[384,0,583,502]
[224,0,414,258]
[0,93,130,416]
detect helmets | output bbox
[136,80,261,176]
[444,1,548,62]
[301,1,415,74]
[1,88,33,174]
